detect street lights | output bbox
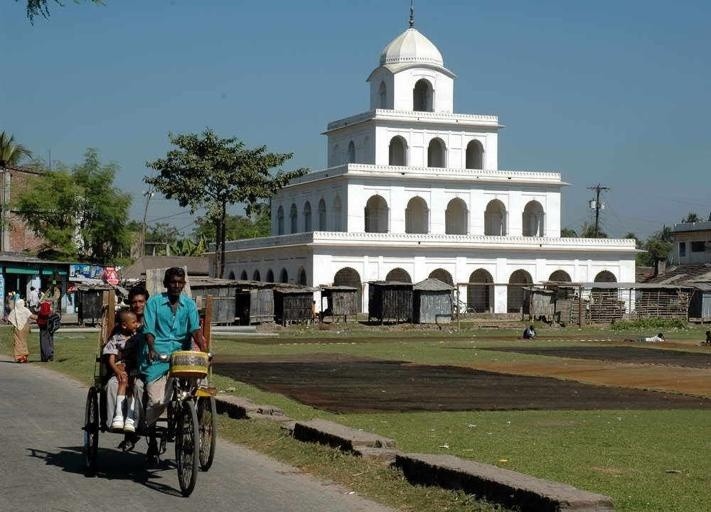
[590,199,605,236]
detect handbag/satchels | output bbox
[47,314,60,333]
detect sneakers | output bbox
[111,415,135,432]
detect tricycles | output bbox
[82,351,217,498]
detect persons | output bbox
[31,287,56,363]
[127,285,151,336]
[134,266,210,468]
[705,330,711,346]
[623,332,667,343]
[101,311,142,434]
[27,285,40,314]
[7,299,39,364]
[523,325,537,340]
